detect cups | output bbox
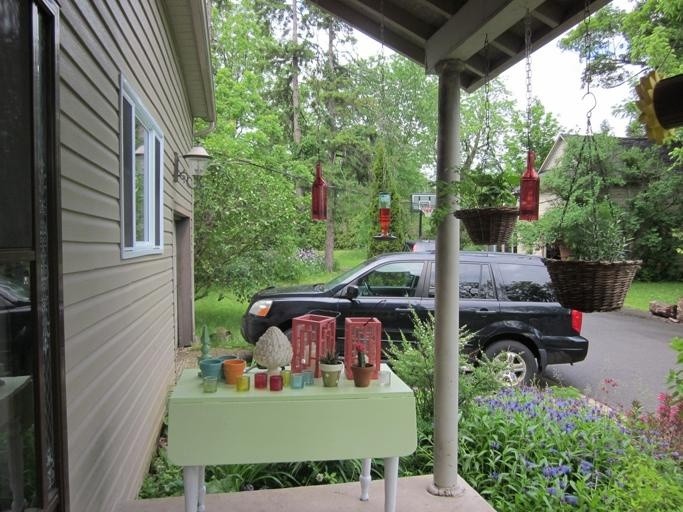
[377,370,391,386]
[198,354,314,394]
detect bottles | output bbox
[311,162,328,220]
[519,149,540,221]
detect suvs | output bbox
[238,249,588,392]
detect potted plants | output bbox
[436,168,521,245]
[543,209,642,312]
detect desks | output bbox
[167,367,416,511]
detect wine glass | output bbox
[373,192,400,240]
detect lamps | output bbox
[172,147,214,190]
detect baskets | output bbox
[451,206,521,246]
[539,254,644,314]
